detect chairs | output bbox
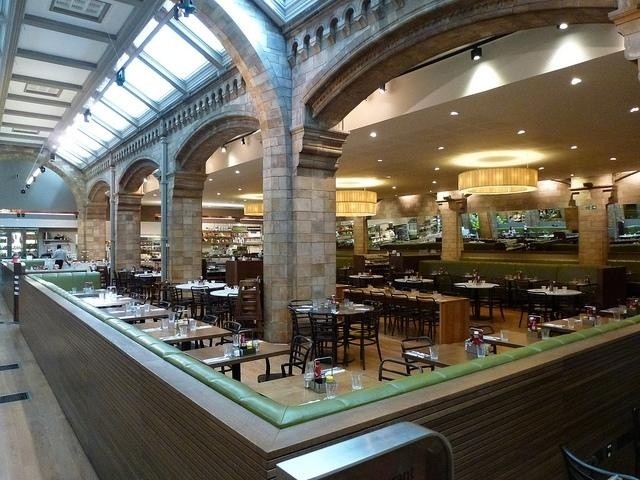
[29,260,109,272]
[558,441,640,480]
[465,271,640,355]
[168,262,291,382]
[336,262,505,381]
[68,265,233,351]
[247,296,382,406]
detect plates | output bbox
[372,289,433,299]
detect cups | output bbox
[505,275,513,280]
[71,287,77,294]
[124,300,150,314]
[225,285,238,293]
[188,280,215,285]
[81,259,94,264]
[402,276,422,282]
[541,328,550,339]
[465,273,472,277]
[568,314,601,328]
[465,338,489,358]
[432,291,443,303]
[541,281,567,293]
[161,313,196,337]
[468,280,485,285]
[350,369,363,390]
[500,330,510,342]
[312,298,353,313]
[411,289,419,297]
[98,291,117,303]
[613,305,626,321]
[83,287,94,294]
[429,346,440,361]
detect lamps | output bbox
[17,0,200,194]
[242,198,264,217]
[221,129,259,153]
[455,159,540,195]
[376,22,572,95]
[335,186,379,217]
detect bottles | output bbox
[233,232,248,243]
[358,269,372,277]
[303,361,336,399]
[473,269,480,283]
[140,240,152,262]
[201,223,232,243]
[223,333,260,357]
[585,272,592,284]
[384,283,395,294]
[331,295,340,314]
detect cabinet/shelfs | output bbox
[140,235,161,272]
[105,241,111,265]
[336,228,354,249]
[0,227,39,259]
[202,227,264,257]
[367,227,396,250]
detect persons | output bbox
[50,244,71,269]
